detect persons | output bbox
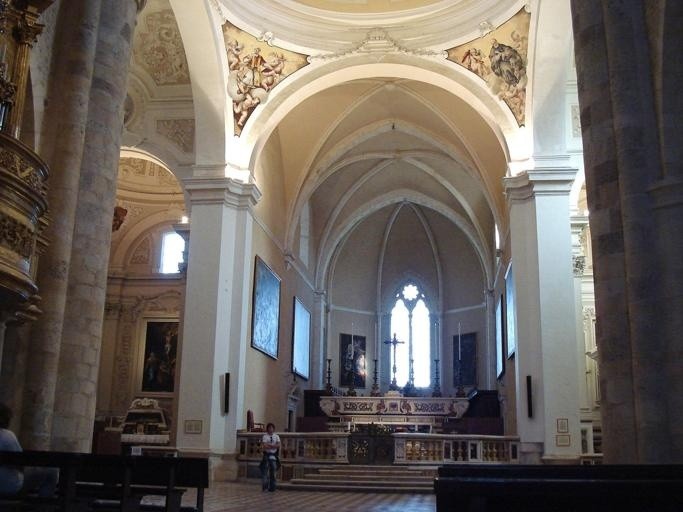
[0,402,59,499]
[258,422,283,491]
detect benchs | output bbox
[0,450,208,512]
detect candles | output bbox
[433,322,438,359]
[350,322,353,356]
[374,321,378,361]
[457,321,461,360]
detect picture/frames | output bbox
[249,255,312,382]
[451,331,478,386]
[129,313,180,397]
[339,332,366,388]
[556,418,570,446]
